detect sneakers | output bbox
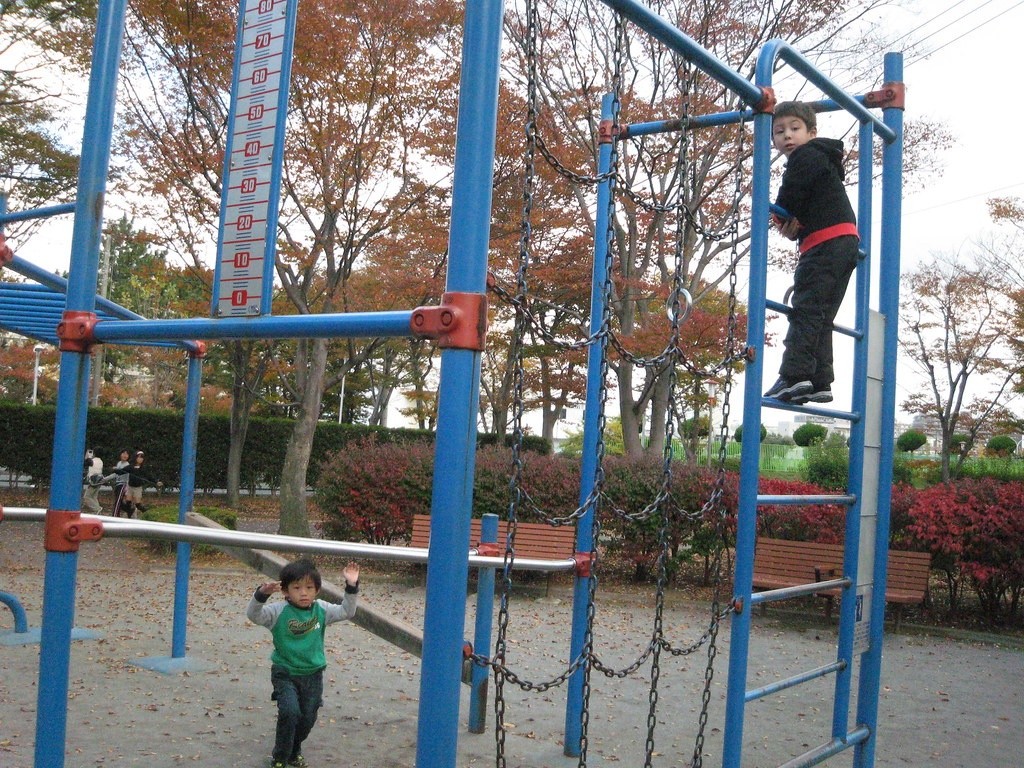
[791,383,833,403]
[763,374,813,403]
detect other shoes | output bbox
[291,753,310,768]
[272,759,288,768]
[93,507,103,515]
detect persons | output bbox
[763,100,861,405]
[104,449,162,519]
[246,559,361,768]
[83,446,104,515]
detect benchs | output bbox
[752,538,931,634]
[409,512,575,597]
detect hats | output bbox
[137,450,144,455]
